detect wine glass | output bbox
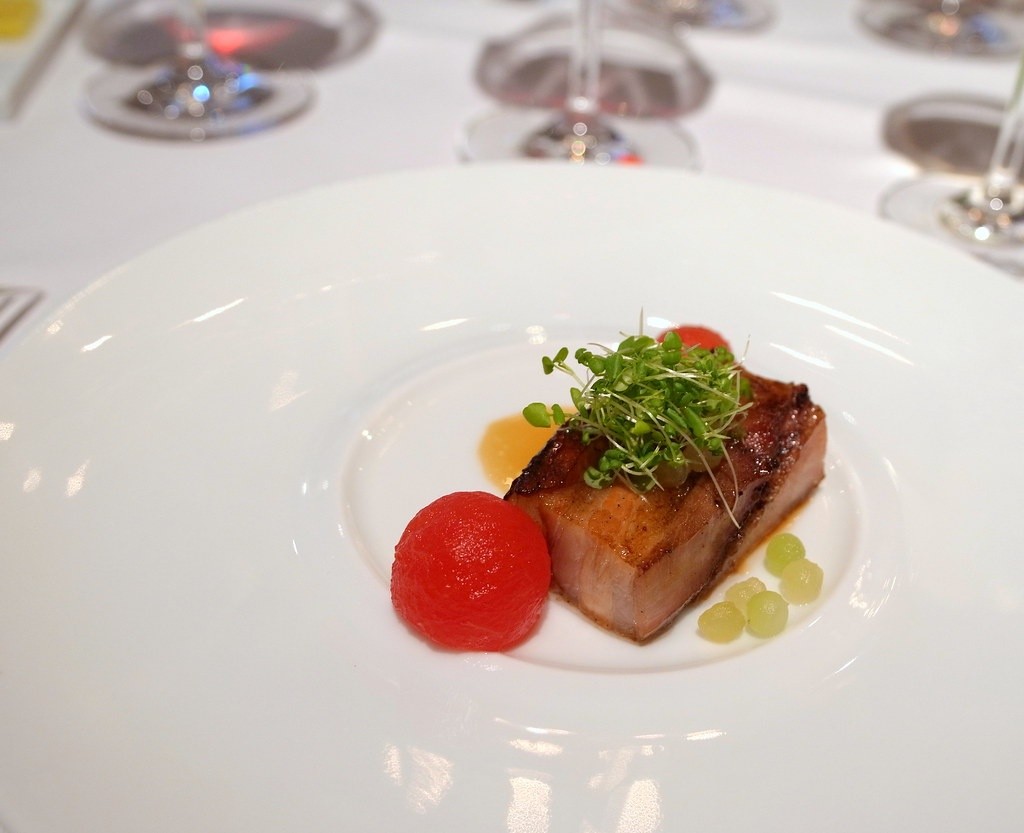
[79,0,1023,287]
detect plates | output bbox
[0,158,1024,833]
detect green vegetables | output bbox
[521,308,752,529]
